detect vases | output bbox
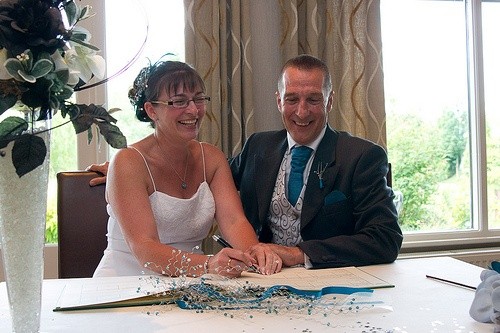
[0,117,54,333]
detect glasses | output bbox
[151,96,211,108]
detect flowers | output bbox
[0,0,128,179]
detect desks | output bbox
[0,256,500,333]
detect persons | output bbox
[93,52,283,280]
[85,54,404,269]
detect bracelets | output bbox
[204,255,212,273]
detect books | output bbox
[52,264,395,313]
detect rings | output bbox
[273,257,278,263]
[228,259,232,267]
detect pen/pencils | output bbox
[212,234,260,274]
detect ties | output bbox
[287,145,313,208]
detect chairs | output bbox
[57,172,107,279]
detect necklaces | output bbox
[171,154,188,188]
